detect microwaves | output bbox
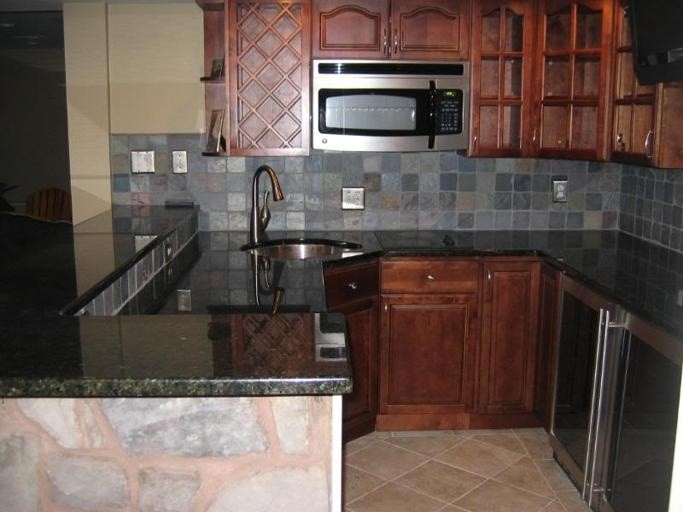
[309,57,472,153]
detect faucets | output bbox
[249,165,285,244]
[250,253,284,317]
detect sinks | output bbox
[240,238,364,262]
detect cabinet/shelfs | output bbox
[548,274,683,511]
[478,257,563,437]
[322,257,378,444]
[378,256,479,430]
